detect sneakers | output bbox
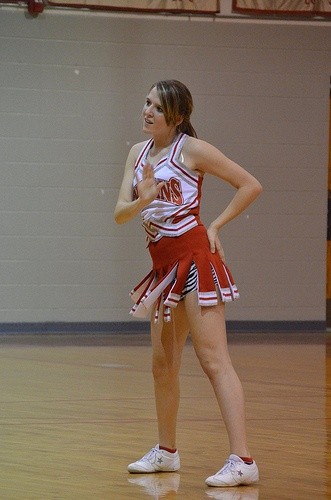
[127,444,181,473]
[205,453,259,486]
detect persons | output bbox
[115,79,266,491]
